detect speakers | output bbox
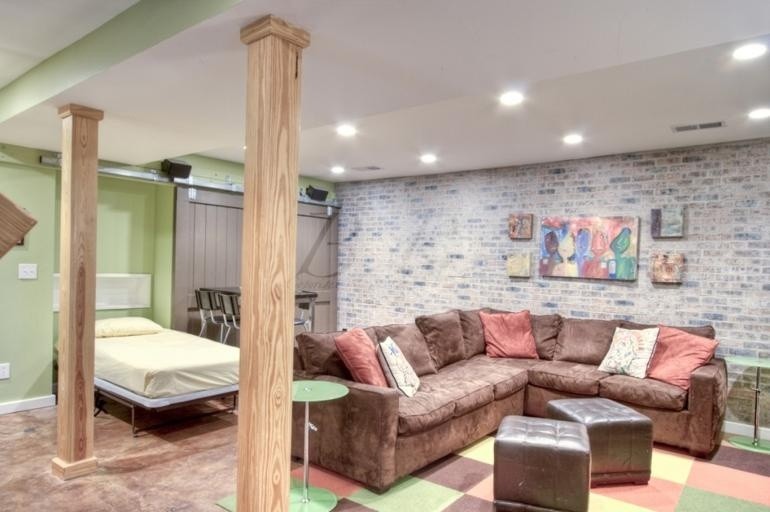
[305,182,328,201]
[161,158,191,180]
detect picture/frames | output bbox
[539,215,641,282]
[650,249,683,284]
[651,208,684,239]
[508,213,533,239]
[508,253,530,278]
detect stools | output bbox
[546,396,652,486]
[492,414,589,512]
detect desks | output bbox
[289,379,349,512]
[725,355,770,455]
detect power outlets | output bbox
[18,262,36,279]
[0,365,12,380]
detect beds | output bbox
[52,272,240,437]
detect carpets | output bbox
[217,414,770,512]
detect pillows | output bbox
[334,326,389,387]
[479,309,540,359]
[93,317,164,337]
[376,335,421,398]
[596,326,660,378]
[649,323,720,391]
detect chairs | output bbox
[218,293,240,344]
[195,290,224,344]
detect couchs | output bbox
[295,307,728,495]
